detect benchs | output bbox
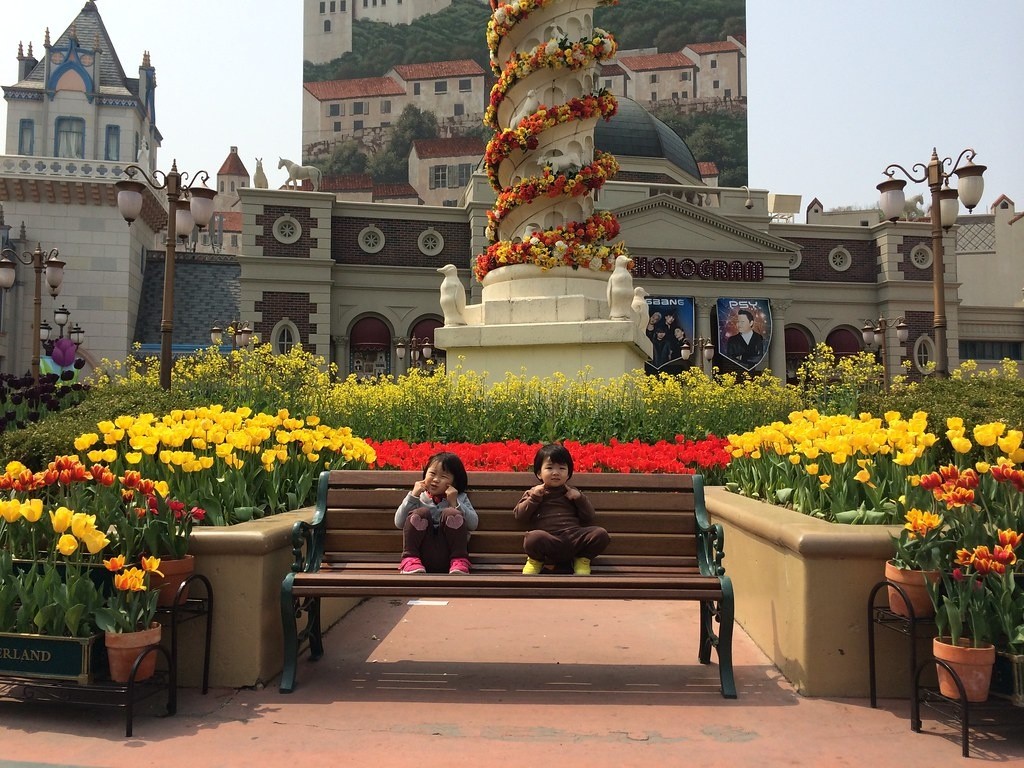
[280,470,737,698]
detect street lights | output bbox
[209,316,251,350]
[860,314,908,391]
[0,242,67,388]
[876,147,987,378]
[395,332,433,369]
[680,333,715,372]
[115,158,219,390]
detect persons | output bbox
[512,443,610,576]
[646,311,693,369]
[726,309,764,370]
[393,452,479,576]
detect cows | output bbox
[510,89,539,129]
[536,152,581,178]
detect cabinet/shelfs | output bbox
[2,574,213,737]
[867,581,1023,757]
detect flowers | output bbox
[0,455,206,635]
[890,462,1024,653]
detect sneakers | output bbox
[573,557,591,576]
[522,557,544,575]
[449,558,474,575]
[397,557,426,574]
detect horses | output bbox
[278,156,322,192]
[253,157,269,189]
[902,193,925,221]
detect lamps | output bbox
[741,185,754,209]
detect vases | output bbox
[1,632,100,686]
[10,550,137,598]
[933,637,995,702]
[945,573,1024,594]
[990,650,1024,706]
[885,560,941,619]
[105,622,161,684]
[148,556,194,604]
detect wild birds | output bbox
[607,255,650,335]
[550,23,565,39]
[436,263,467,326]
[522,225,536,241]
